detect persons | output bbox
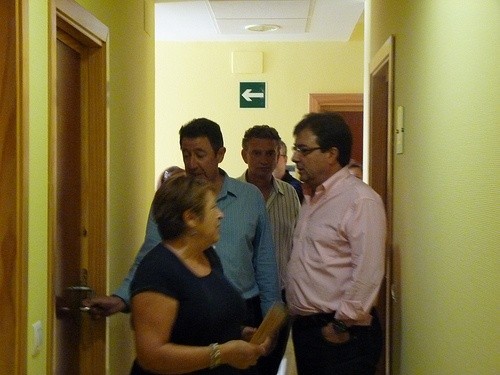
[235,125,303,375]
[284,111,386,375]
[127,175,271,375]
[80,117,282,356]
[272,140,362,206]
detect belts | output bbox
[294,316,333,330]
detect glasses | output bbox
[291,146,325,156]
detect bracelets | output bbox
[332,317,352,332]
[209,342,223,368]
